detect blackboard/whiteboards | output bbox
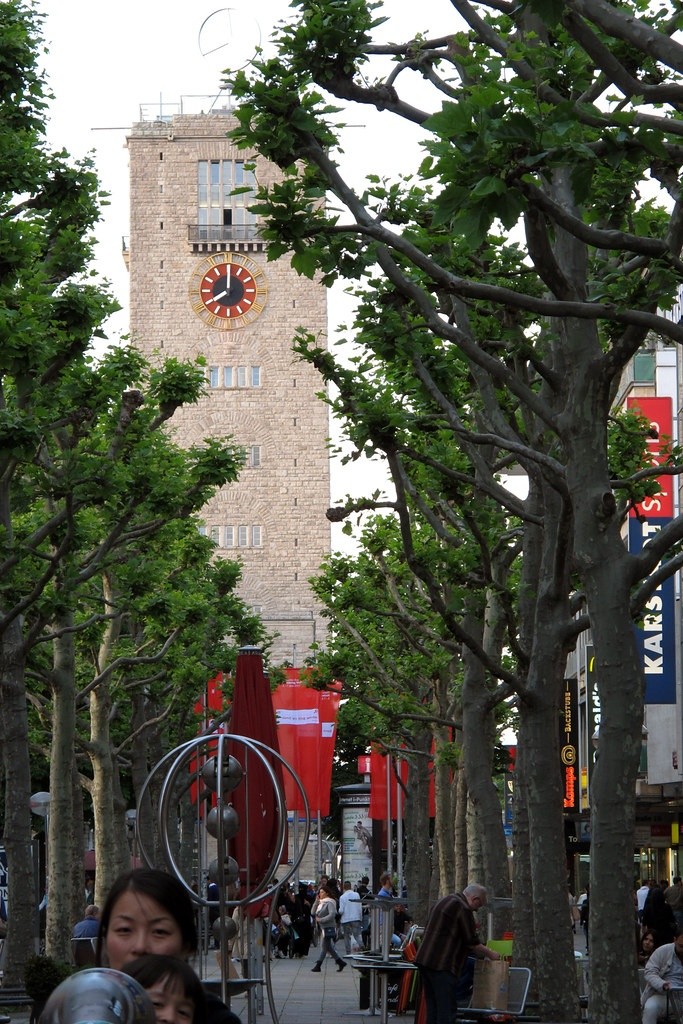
[378,973,402,1012]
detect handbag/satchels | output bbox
[231,957,243,979]
[471,959,510,1011]
[290,926,299,939]
[350,933,362,949]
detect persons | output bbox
[121,953,205,1024]
[72,905,100,938]
[95,868,242,1024]
[85,878,94,905]
[354,821,372,856]
[206,875,374,997]
[414,885,500,1024]
[569,882,589,955]
[637,876,683,1024]
[377,873,413,948]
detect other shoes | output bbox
[278,952,286,959]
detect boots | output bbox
[311,960,323,972]
[335,958,347,972]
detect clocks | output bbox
[187,252,268,330]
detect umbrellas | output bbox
[224,644,289,1013]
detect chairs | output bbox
[456,959,647,1024]
